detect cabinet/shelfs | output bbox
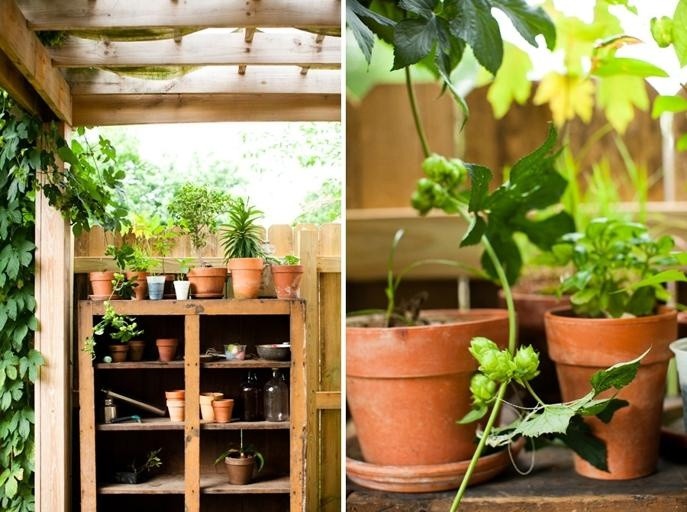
[78,302,300,512]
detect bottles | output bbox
[242,368,264,421]
[263,367,289,423]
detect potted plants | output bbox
[346,230,520,468]
[496,129,663,396]
[213,428,266,485]
[540,217,685,483]
[99,446,163,484]
[90,180,301,301]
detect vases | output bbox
[99,331,290,427]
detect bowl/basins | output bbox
[255,344,291,361]
